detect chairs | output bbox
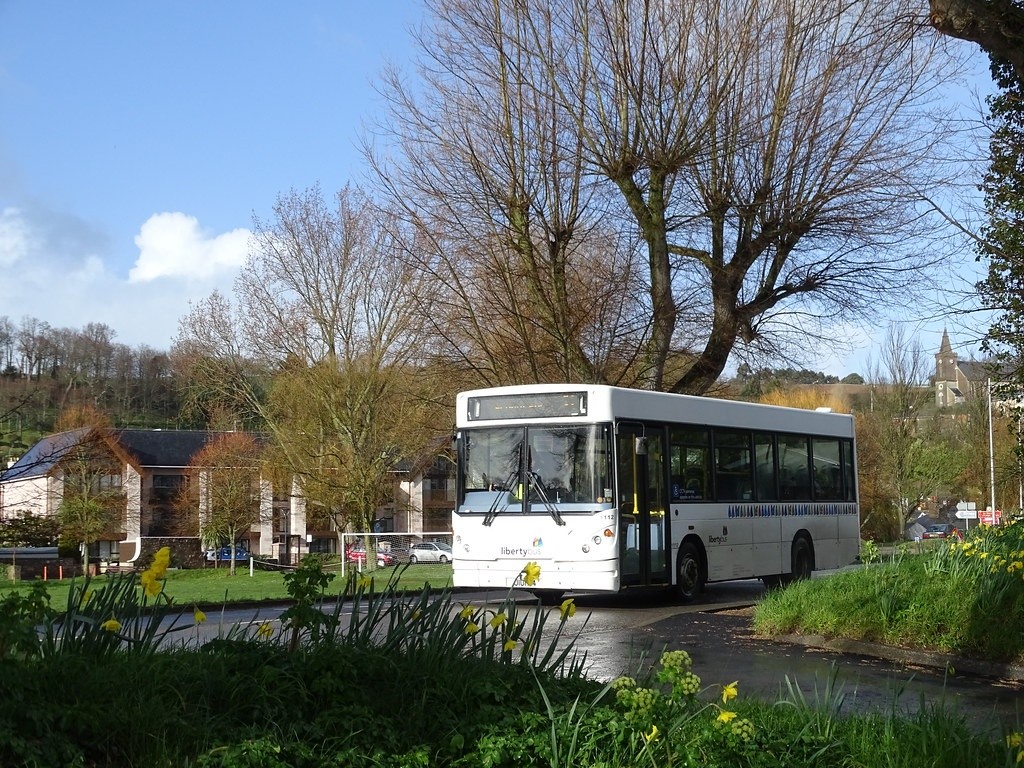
[672,466,849,500]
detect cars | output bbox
[407,542,452,564]
[346,544,398,567]
[205,547,249,562]
[922,522,960,544]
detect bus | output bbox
[445,383,863,601]
